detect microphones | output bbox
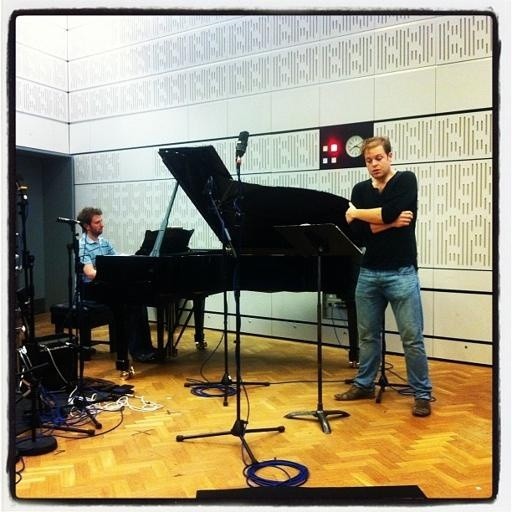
[202,177,211,195]
[236,132,249,157]
[57,217,80,224]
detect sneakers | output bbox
[334,384,376,401]
[130,345,167,365]
[411,394,432,418]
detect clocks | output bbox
[346,136,364,157]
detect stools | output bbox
[49,301,115,361]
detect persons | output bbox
[334,136,433,417]
[74,206,167,364]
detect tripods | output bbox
[15,224,118,435]
[285,248,349,434]
[345,296,411,404]
[177,157,285,467]
[184,192,270,407]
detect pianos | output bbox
[95,147,358,369]
